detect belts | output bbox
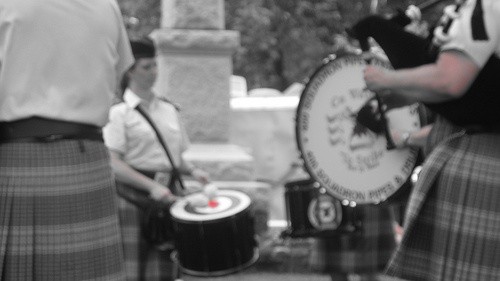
[0,116,104,142]
[138,170,174,188]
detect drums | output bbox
[167,188,260,278]
[277,178,366,240]
[292,44,423,209]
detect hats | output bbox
[129,35,155,57]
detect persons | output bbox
[365,0,500,281]
[1,0,136,281]
[310,201,397,281]
[103,35,211,281]
